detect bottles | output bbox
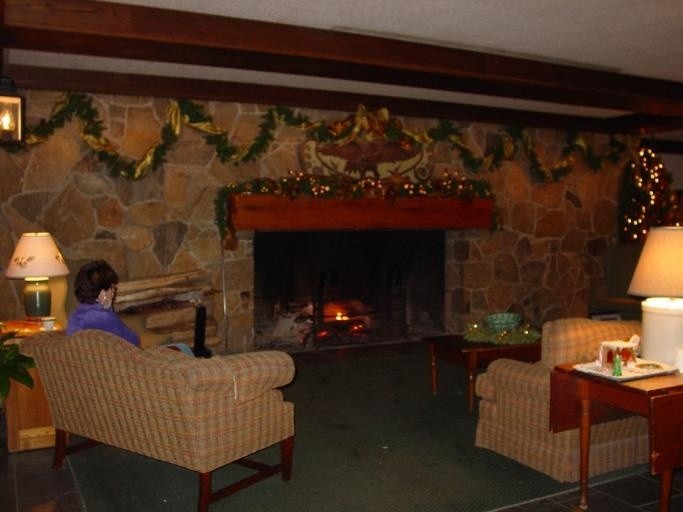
[613,353,624,376]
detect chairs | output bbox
[474,317,650,483]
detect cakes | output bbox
[597,336,640,375]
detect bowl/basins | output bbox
[484,312,524,330]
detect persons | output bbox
[66,259,195,359]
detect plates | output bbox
[38,327,57,332]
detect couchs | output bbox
[28,330,296,512]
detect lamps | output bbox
[0,76,25,148]
[626,227,683,301]
[4,232,70,318]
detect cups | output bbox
[41,317,57,330]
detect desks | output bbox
[549,362,683,512]
[421,336,541,412]
[0,318,70,453]
